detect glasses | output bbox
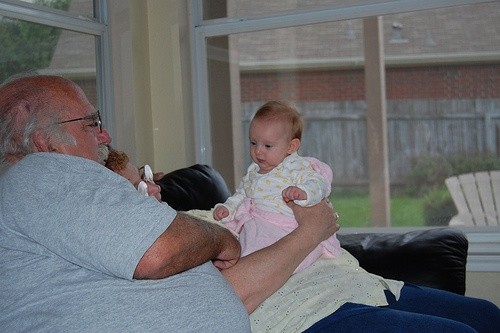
[44,110,102,134]
[137,164,153,197]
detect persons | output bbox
[0,72,252,333]
[212,101,341,276]
[105,150,500,333]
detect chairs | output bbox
[445,171,500,226]
[157,163,468,295]
